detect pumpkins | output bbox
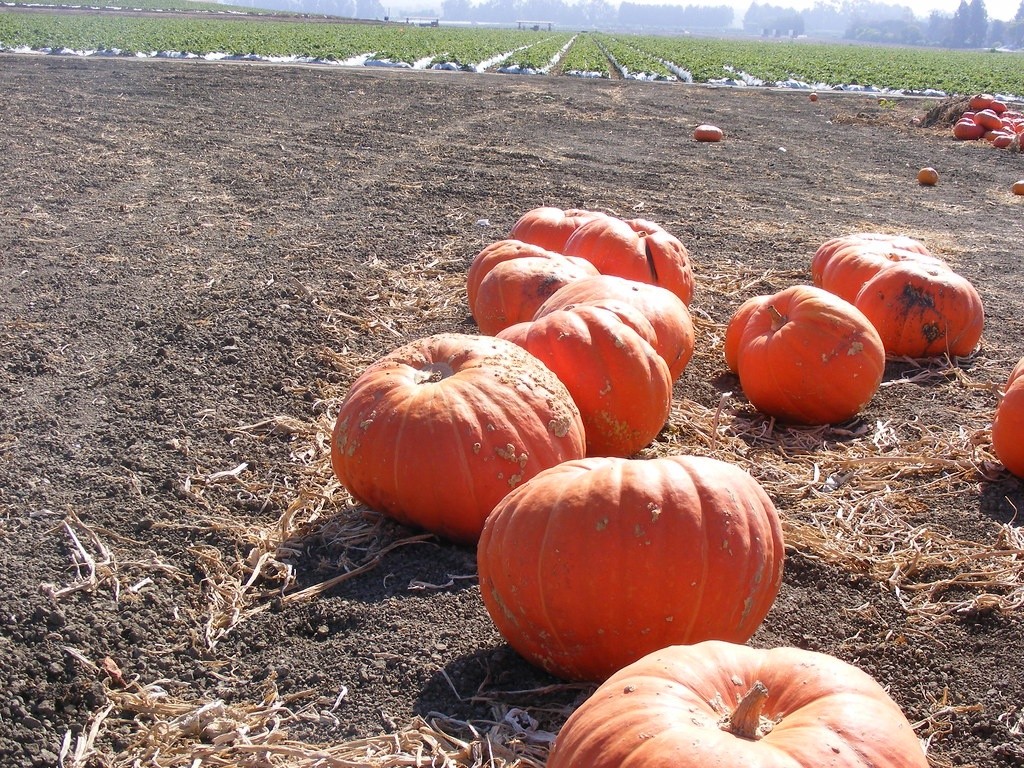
[810,93,818,101]
[1013,181,1024,195]
[464,205,986,460]
[991,356,1024,478]
[546,640,933,768]
[953,94,1024,151]
[695,125,723,142]
[331,332,586,543]
[476,455,785,683]
[918,168,938,185]
